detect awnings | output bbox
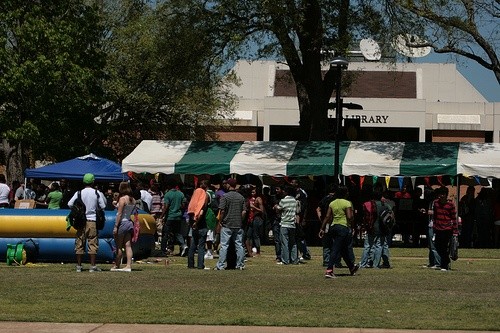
[122,139,500,180]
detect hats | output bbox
[83,173,95,183]
[225,178,237,188]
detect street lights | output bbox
[329,54,348,184]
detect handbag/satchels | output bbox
[96,208,106,230]
[253,205,268,229]
[450,235,459,260]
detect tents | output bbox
[24,153,130,182]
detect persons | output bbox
[110,183,136,272]
[187,180,248,270]
[67,174,107,272]
[417,187,459,272]
[316,183,395,268]
[308,180,500,249]
[318,185,361,278]
[266,180,311,264]
[0,173,274,256]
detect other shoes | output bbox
[324,272,337,278]
[299,256,304,259]
[440,269,447,272]
[76,265,84,272]
[180,245,189,257]
[89,265,104,273]
[165,248,173,255]
[276,261,285,264]
[421,264,441,269]
[155,252,168,257]
[204,253,214,259]
[351,264,360,275]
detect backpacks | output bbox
[379,209,396,233]
[69,191,87,230]
